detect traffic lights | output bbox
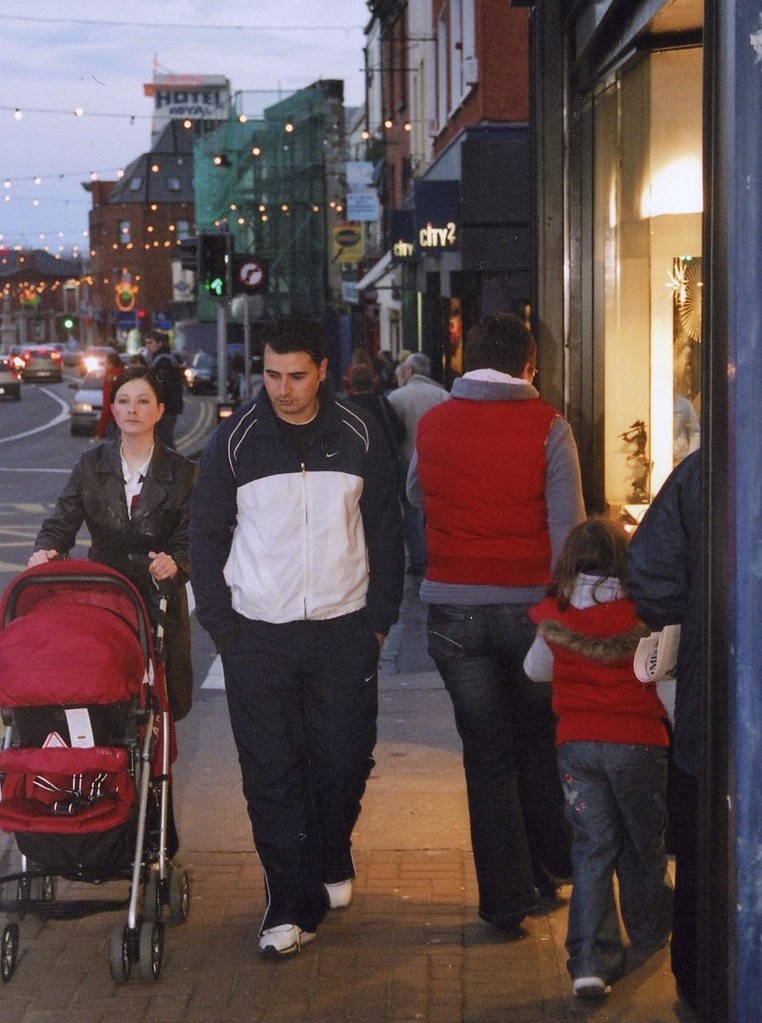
[64,315,76,332]
[178,235,202,275]
[203,232,234,300]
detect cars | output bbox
[189,352,239,396]
[66,347,149,438]
[0,343,84,400]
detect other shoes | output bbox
[573,977,606,999]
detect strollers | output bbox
[0,553,191,985]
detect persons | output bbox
[27,314,706,999]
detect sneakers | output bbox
[323,878,353,910]
[257,923,316,959]
